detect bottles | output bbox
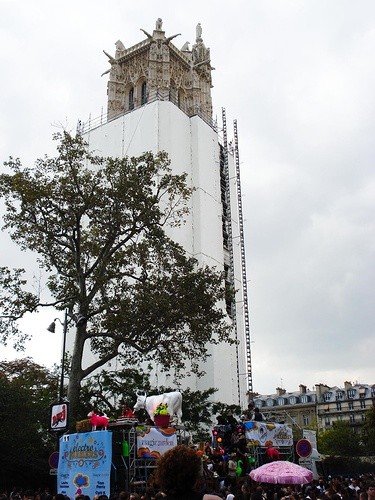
[122,401,132,417]
[138,407,150,422]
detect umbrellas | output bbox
[250,461,314,484]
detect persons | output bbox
[0,486,167,500]
[158,445,223,500]
[196,441,251,483]
[122,404,154,425]
[217,404,276,425]
[208,475,375,500]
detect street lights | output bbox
[45,308,87,455]
